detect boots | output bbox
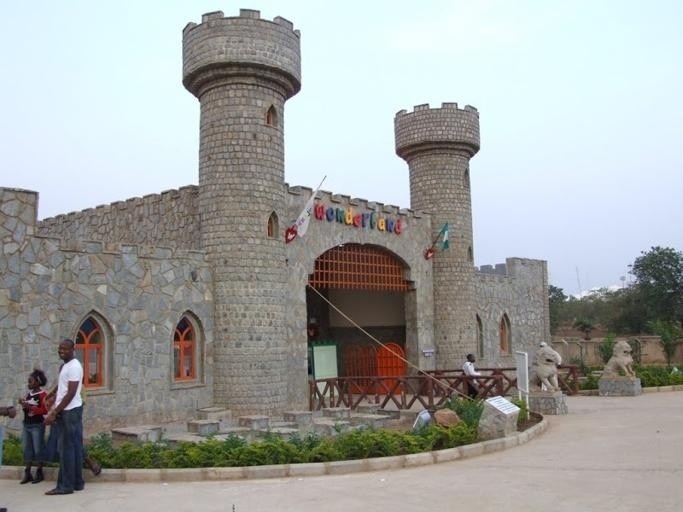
[19,468,44,484]
[84,457,101,475]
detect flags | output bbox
[441,222,450,253]
[294,175,327,237]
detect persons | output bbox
[19,339,100,495]
[0,406,16,512]
[463,354,481,401]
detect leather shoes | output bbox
[44,486,84,495]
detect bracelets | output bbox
[4,408,9,416]
